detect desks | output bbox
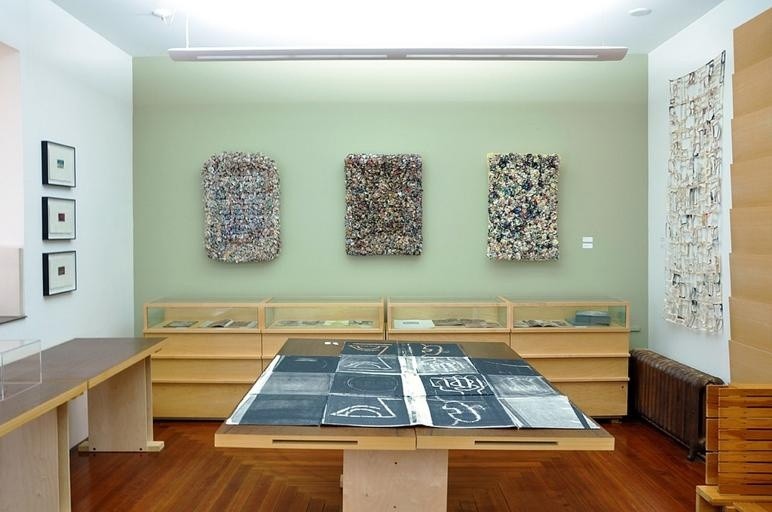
[214,336,615,512]
[1,335,171,511]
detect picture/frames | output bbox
[41,141,77,296]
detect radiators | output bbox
[625,347,724,460]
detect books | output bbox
[348,318,375,328]
[394,309,580,329]
[163,317,200,327]
[271,317,298,326]
[201,317,259,328]
[299,317,349,327]
